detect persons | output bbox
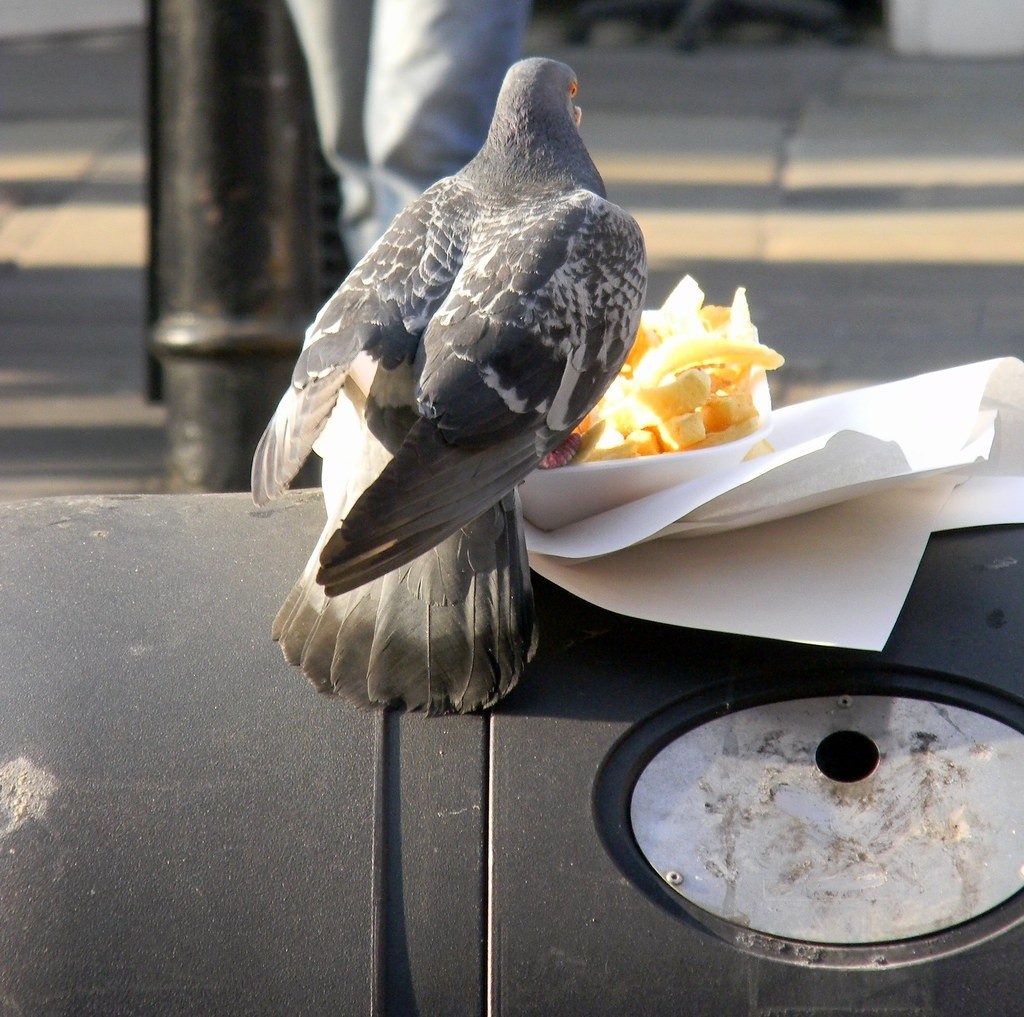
[288,2,528,268]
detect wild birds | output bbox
[251,55,650,716]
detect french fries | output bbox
[569,274,787,462]
